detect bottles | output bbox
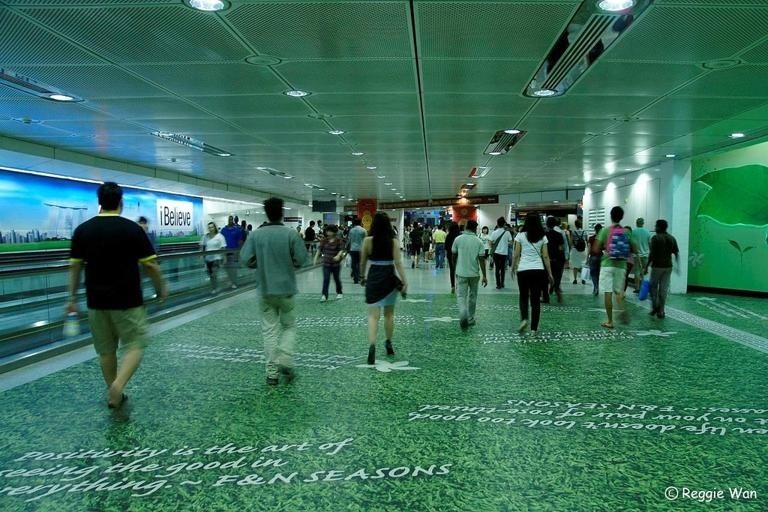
[64,310,81,337]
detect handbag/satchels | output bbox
[490,243,498,256]
[580,265,590,282]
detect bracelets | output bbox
[68,295,78,301]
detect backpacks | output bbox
[574,231,585,252]
[609,225,630,261]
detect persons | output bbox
[359,209,409,365]
[241,197,307,385]
[137,216,156,253]
[200,205,681,337]
[61,180,169,410]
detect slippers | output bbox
[108,394,127,410]
